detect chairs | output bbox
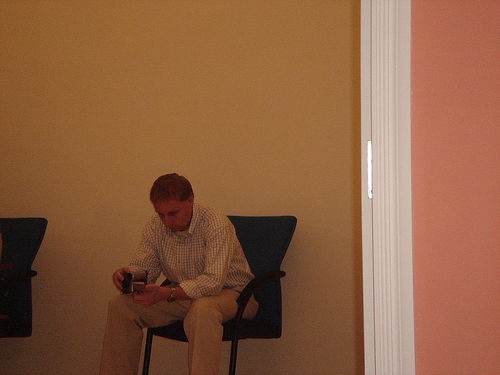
[142,215,297,375]
[0,217,48,337]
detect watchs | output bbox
[167,287,177,303]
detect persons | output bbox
[99,173,259,375]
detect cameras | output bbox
[122,269,148,296]
